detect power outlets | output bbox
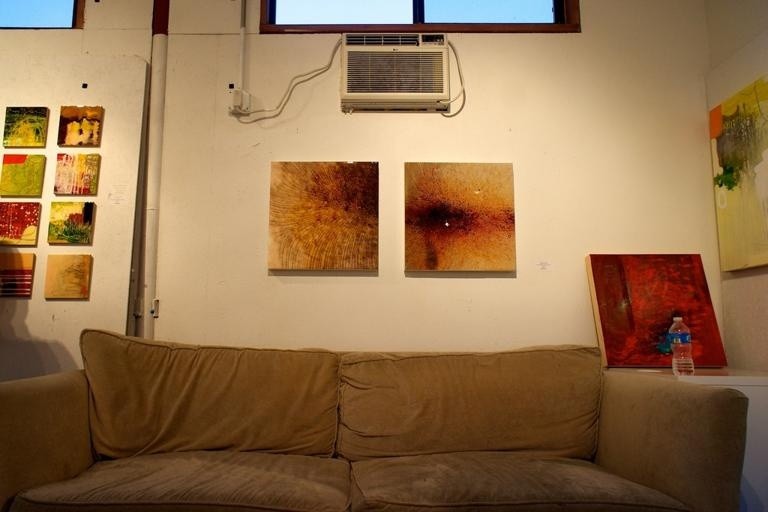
[222,87,249,113]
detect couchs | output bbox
[0,327,748,512]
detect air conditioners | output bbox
[339,32,452,113]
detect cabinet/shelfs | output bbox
[642,364,766,481]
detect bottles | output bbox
[668,316,695,376]
